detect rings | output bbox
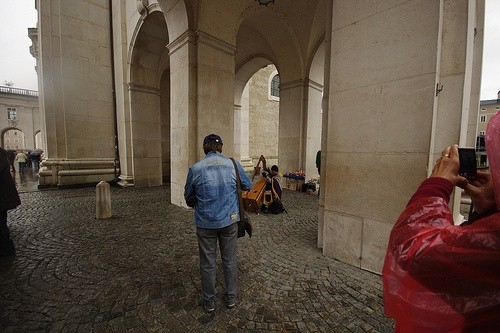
[441,154,449,158]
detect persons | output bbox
[382,109,500,333]
[0,147,22,254]
[6,149,42,178]
[263,165,282,214]
[184,134,253,312]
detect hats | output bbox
[203,134,223,144]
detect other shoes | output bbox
[202,299,215,311]
[0,240,15,255]
[226,294,235,306]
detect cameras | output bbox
[457,148,478,182]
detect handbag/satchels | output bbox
[237,210,253,238]
[270,198,284,214]
